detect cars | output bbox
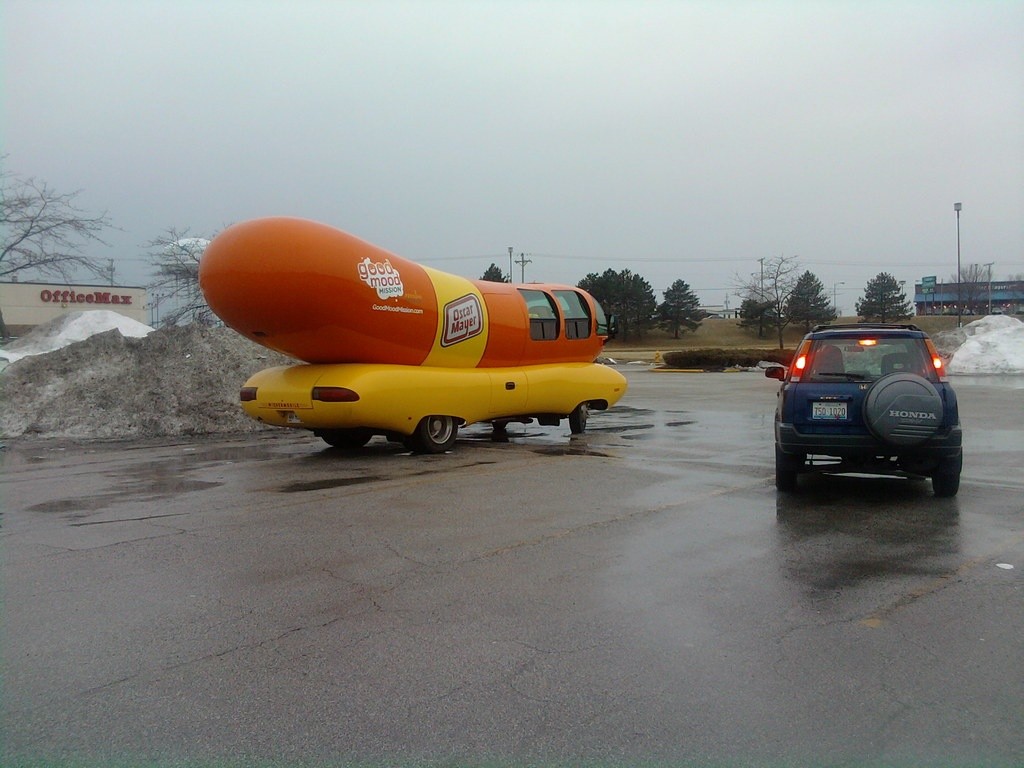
[992,308,1002,314]
[964,310,974,315]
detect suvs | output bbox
[765,323,963,495]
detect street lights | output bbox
[508,247,514,283]
[953,202,961,326]
[833,281,844,309]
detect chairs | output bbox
[815,345,844,378]
[881,353,919,377]
